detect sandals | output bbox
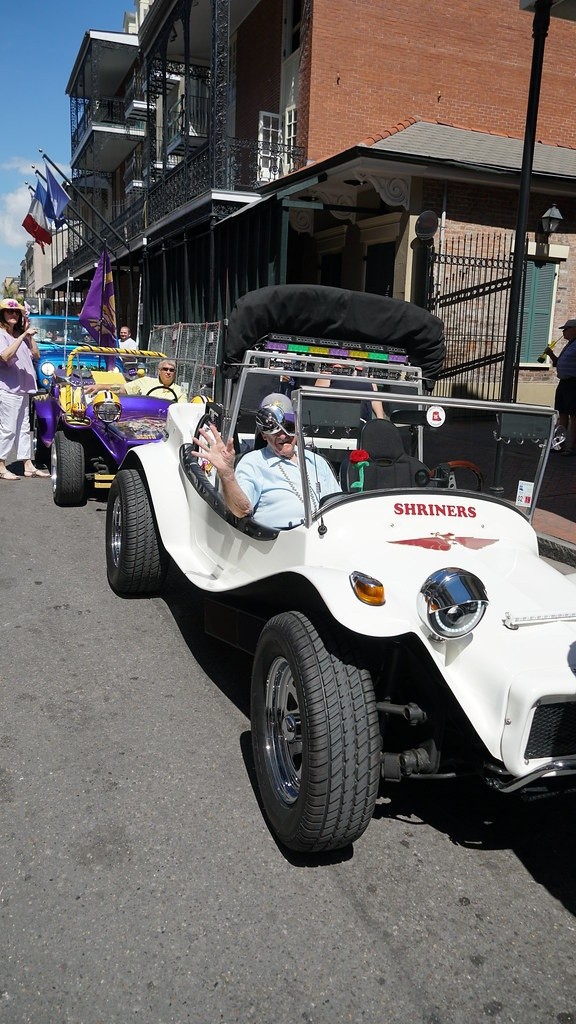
[24,469,50,478]
[0,471,19,480]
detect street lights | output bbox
[20,288,28,301]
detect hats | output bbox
[0,299,25,316]
[261,392,294,422]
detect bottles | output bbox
[537,341,557,364]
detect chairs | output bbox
[341,419,433,493]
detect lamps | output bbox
[169,26,178,42]
[541,203,563,243]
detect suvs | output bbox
[28,305,149,389]
[30,343,215,506]
[105,282,576,854]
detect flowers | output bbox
[348,450,371,493]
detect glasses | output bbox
[161,367,175,372]
[4,309,21,315]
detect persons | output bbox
[46,327,62,341]
[84,360,187,403]
[81,326,91,342]
[545,319,576,428]
[314,370,384,423]
[0,299,51,480]
[191,393,342,528]
[118,326,137,373]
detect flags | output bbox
[22,180,56,255]
[78,251,117,372]
[43,166,72,227]
